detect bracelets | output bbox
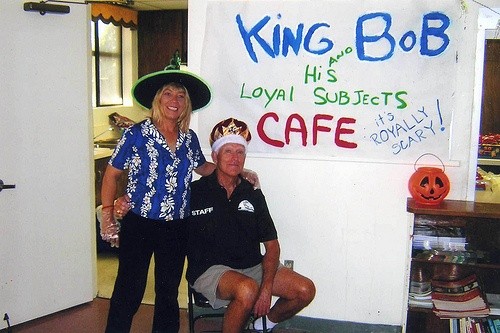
[101,204,114,209]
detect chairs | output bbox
[187,275,266,333]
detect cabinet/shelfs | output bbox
[401,197,500,333]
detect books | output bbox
[408,214,500,333]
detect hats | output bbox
[209,118,251,152]
[131,49,213,113]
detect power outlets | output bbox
[284,260,293,272]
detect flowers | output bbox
[478,133,500,159]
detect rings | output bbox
[116,209,120,213]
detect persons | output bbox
[113,118,317,333]
[100,50,260,333]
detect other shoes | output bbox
[242,312,272,333]
